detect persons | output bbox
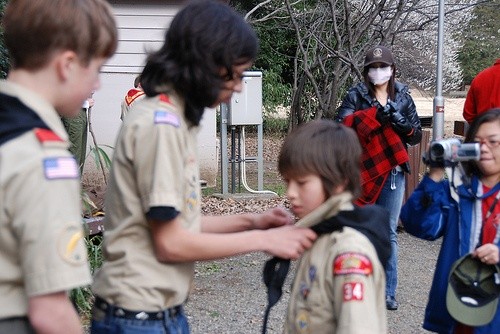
[279,119,386,334]
[0,0,118,334]
[61,76,146,174]
[400,109,500,334]
[339,47,422,308]
[89,0,318,334]
[462,59,500,124]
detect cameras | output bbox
[382,101,406,124]
[429,138,480,166]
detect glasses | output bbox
[471,134,500,148]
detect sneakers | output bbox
[386,295,398,310]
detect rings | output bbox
[484,257,488,261]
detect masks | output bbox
[367,65,393,85]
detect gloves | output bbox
[374,109,388,128]
[391,113,412,135]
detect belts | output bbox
[95,296,181,321]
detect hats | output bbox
[364,46,393,66]
[446,251,500,327]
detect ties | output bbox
[261,202,391,334]
[0,93,50,146]
